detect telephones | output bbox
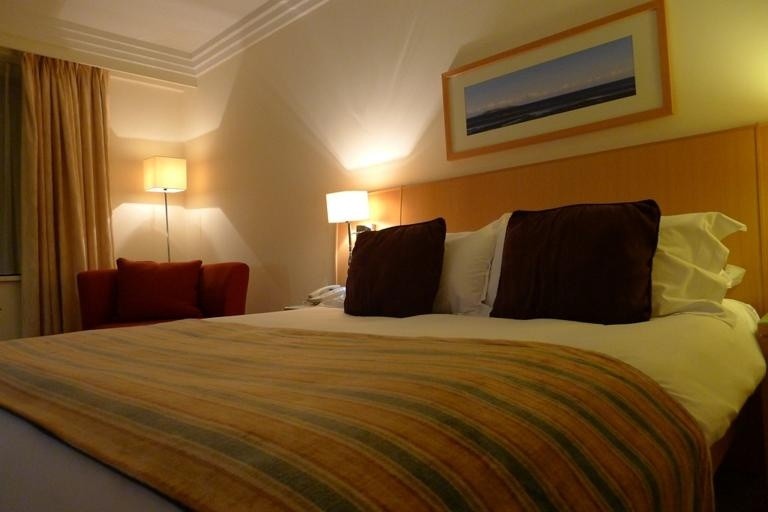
[307,285,346,302]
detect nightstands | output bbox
[285,305,315,310]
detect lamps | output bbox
[143,157,187,262]
[326,190,370,265]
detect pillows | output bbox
[343,217,448,319]
[371,220,494,316]
[489,200,745,323]
[116,258,205,321]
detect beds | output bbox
[0,123,768,512]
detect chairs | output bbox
[77,262,250,331]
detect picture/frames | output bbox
[442,0,674,161]
